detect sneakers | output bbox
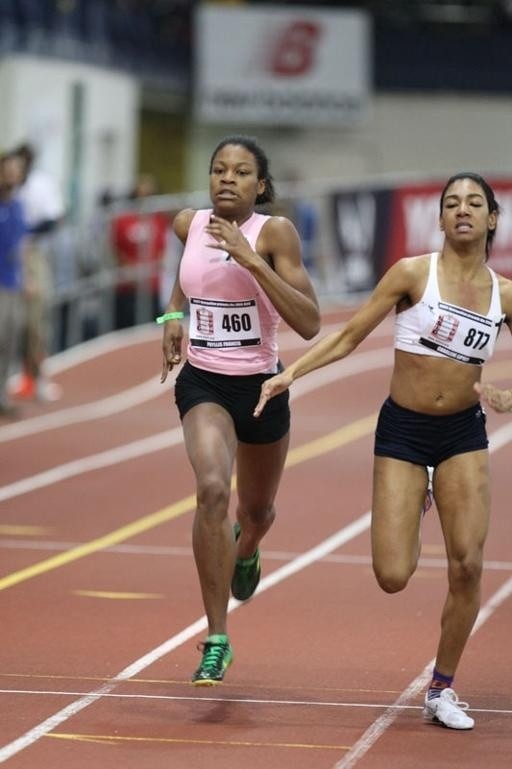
[421,687,476,730]
[229,546,263,603]
[425,464,434,506]
[189,636,233,688]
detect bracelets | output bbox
[155,311,185,324]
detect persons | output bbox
[161,136,321,686]
[1,136,63,418]
[113,167,170,330]
[253,170,512,730]
